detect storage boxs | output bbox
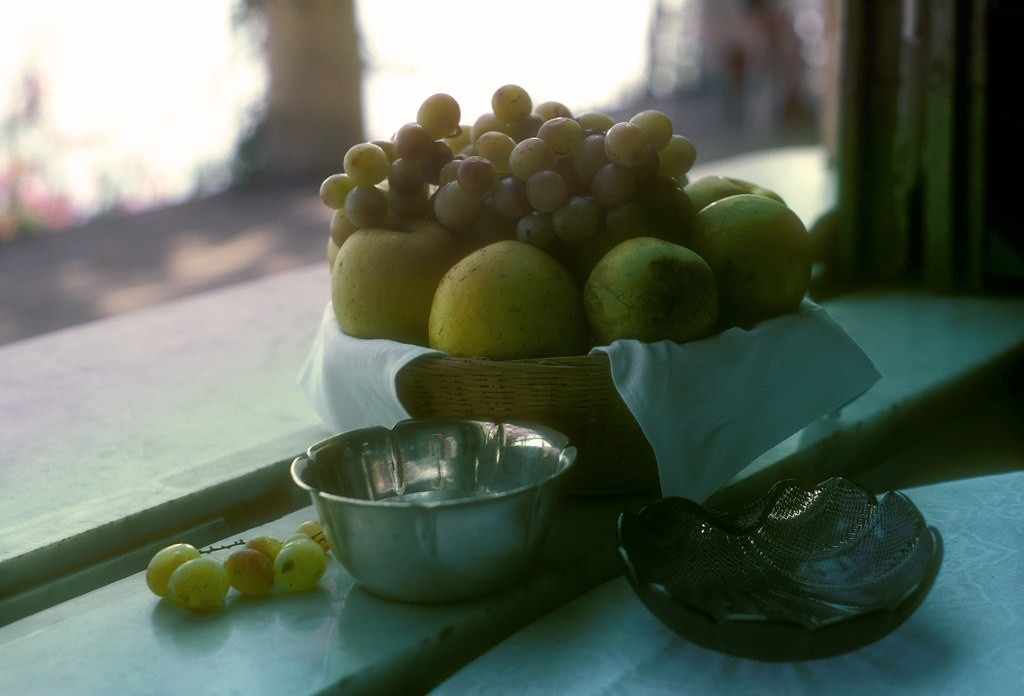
[327,306,810,510]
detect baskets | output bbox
[326,294,844,499]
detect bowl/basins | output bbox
[615,479,945,662]
[291,416,578,599]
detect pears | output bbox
[326,175,815,355]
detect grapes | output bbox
[147,518,331,606]
[315,80,698,240]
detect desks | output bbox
[2,148,1023,696]
